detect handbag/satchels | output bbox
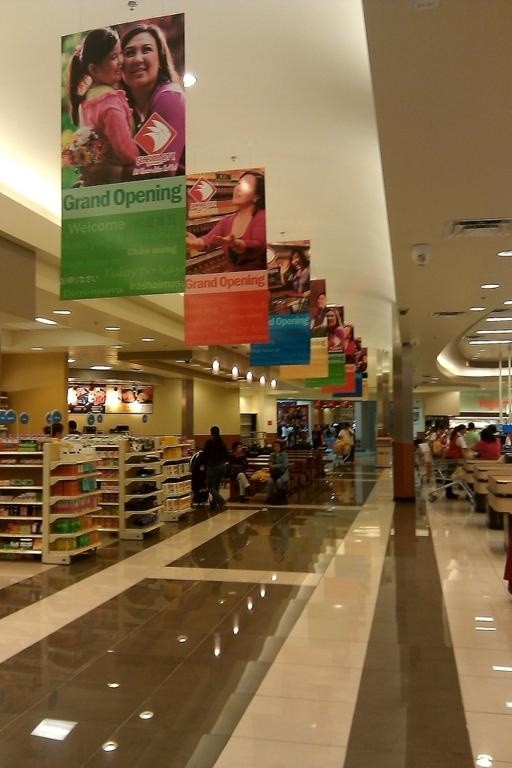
[446,446,463,459]
[270,468,285,479]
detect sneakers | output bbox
[446,489,460,500]
[239,494,246,503]
[246,485,255,496]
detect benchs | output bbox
[220,450,326,502]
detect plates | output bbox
[123,388,136,403]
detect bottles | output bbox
[1,430,51,453]
[51,463,99,548]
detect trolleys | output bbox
[332,440,356,468]
[419,455,477,506]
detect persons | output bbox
[42,426,53,439]
[269,440,291,497]
[203,424,228,507]
[185,171,267,272]
[119,23,185,180]
[227,440,255,503]
[65,27,140,182]
[278,420,355,462]
[422,420,511,501]
[51,422,63,440]
[68,419,81,434]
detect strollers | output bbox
[189,449,212,508]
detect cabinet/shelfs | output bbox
[0,425,199,567]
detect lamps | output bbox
[209,358,277,390]
